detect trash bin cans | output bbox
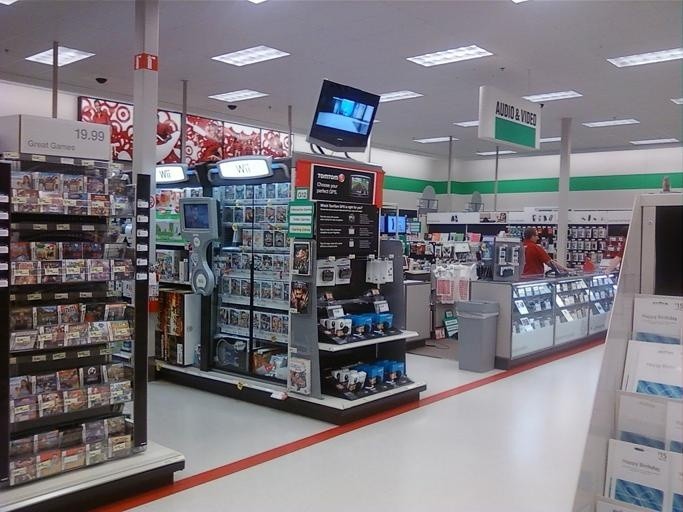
[455,300,500,372]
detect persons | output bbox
[15,379,30,398]
[217,184,288,333]
[519,226,573,275]
[16,174,30,190]
[12,312,29,331]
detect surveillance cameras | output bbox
[96,78,107,83]
[229,106,236,109]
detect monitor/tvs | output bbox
[387,214,406,234]
[305,79,381,152]
[179,197,220,240]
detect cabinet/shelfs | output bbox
[588,271,621,340]
[1,147,184,512]
[468,276,556,367]
[553,273,590,348]
[150,148,430,427]
[378,205,629,282]
[570,190,683,512]
